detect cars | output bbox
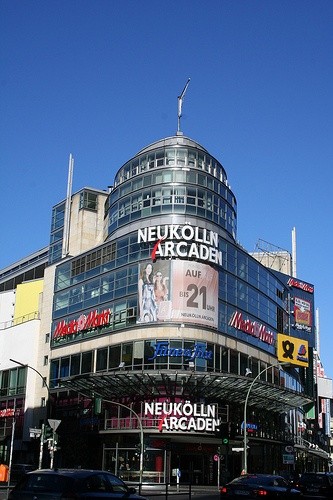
[6,468,150,500]
[297,472,333,500]
[219,473,305,500]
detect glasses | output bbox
[156,275,162,277]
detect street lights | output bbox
[244,362,291,473]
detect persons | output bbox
[138,263,157,322]
[241,469,245,475]
[155,271,169,304]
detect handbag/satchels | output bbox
[157,299,173,320]
